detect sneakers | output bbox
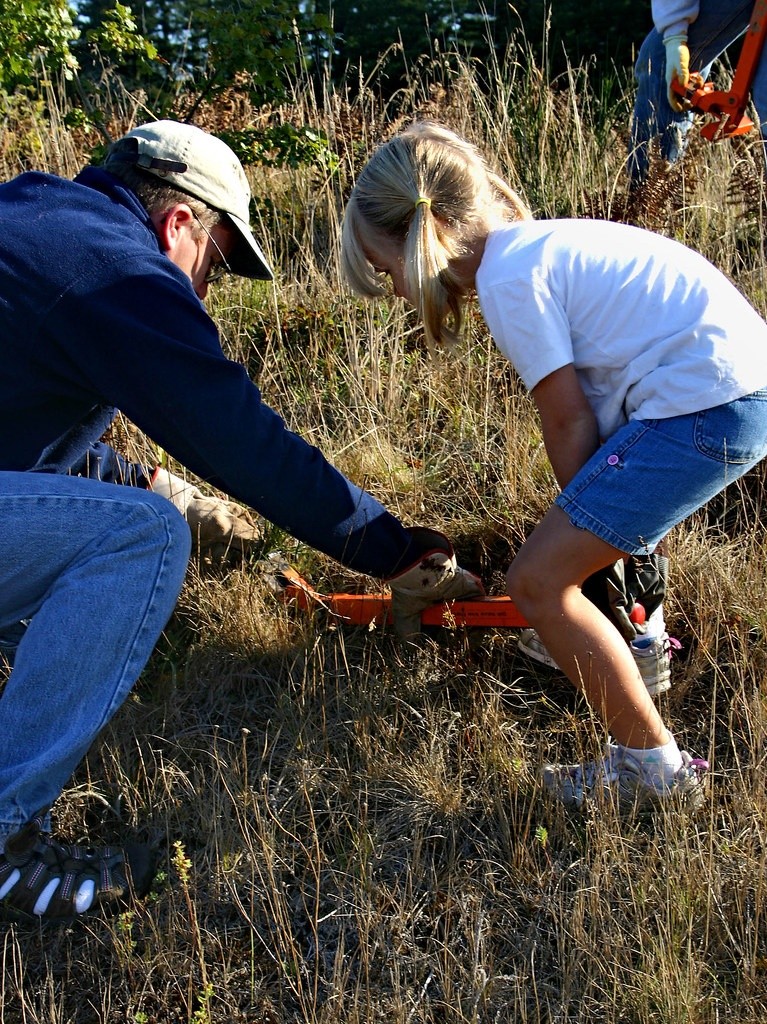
[516,629,683,696]
[543,735,709,815]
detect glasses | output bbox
[160,201,232,284]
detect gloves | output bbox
[662,34,694,113]
[144,464,262,557]
[625,553,669,623]
[381,526,489,655]
[581,557,638,649]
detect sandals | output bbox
[0,804,156,932]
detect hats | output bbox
[104,120,274,280]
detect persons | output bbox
[0,116,484,927]
[630,0,767,217]
[333,127,767,815]
